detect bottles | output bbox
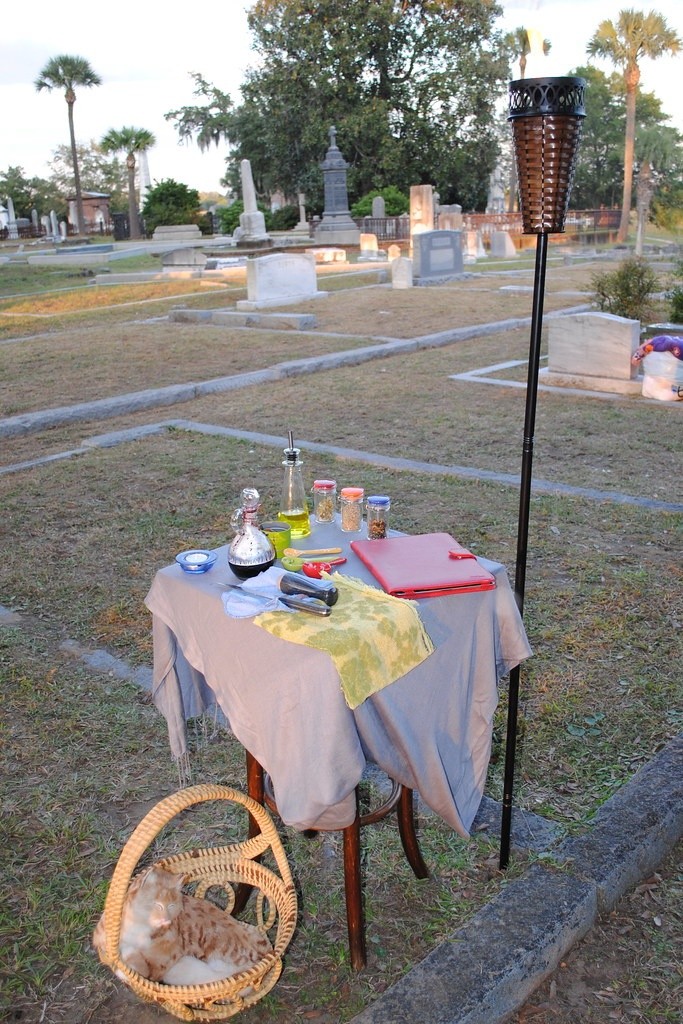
[312,478,338,524]
[339,487,365,533]
[363,495,390,541]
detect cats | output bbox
[91,862,275,1009]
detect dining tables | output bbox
[149,507,510,974]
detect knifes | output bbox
[218,582,331,618]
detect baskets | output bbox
[95,784,300,1022]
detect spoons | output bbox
[283,547,342,558]
[281,555,339,571]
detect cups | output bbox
[259,522,291,559]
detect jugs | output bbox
[227,486,276,580]
[277,446,311,539]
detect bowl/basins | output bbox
[176,549,218,573]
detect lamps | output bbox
[508,75,587,237]
